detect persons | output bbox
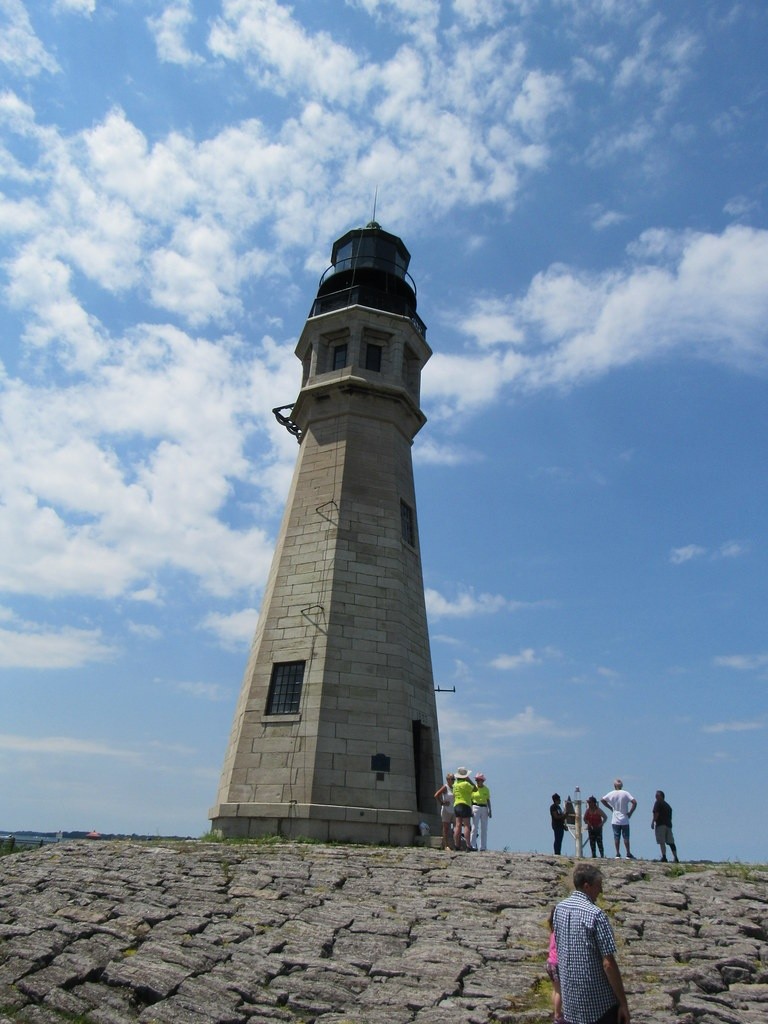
[583,795,607,858]
[469,773,492,851]
[651,790,680,863]
[434,773,464,851]
[452,767,478,851]
[549,793,568,855]
[546,906,568,1024]
[601,779,637,860]
[552,863,631,1024]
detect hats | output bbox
[454,766,471,779]
[473,771,487,782]
[586,796,597,803]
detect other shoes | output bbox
[659,857,667,862]
[441,843,487,852]
[615,853,621,858]
[625,853,636,859]
[554,1010,569,1022]
